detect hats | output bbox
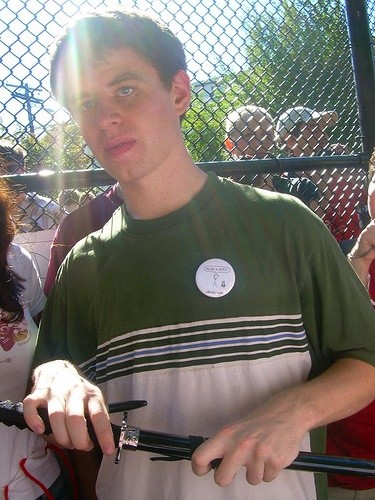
[276,106,338,139]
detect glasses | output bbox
[0,165,24,174]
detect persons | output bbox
[0,106,369,243]
[42,183,124,500]
[326,157,375,500]
[22,12,375,500]
[0,161,66,500]
